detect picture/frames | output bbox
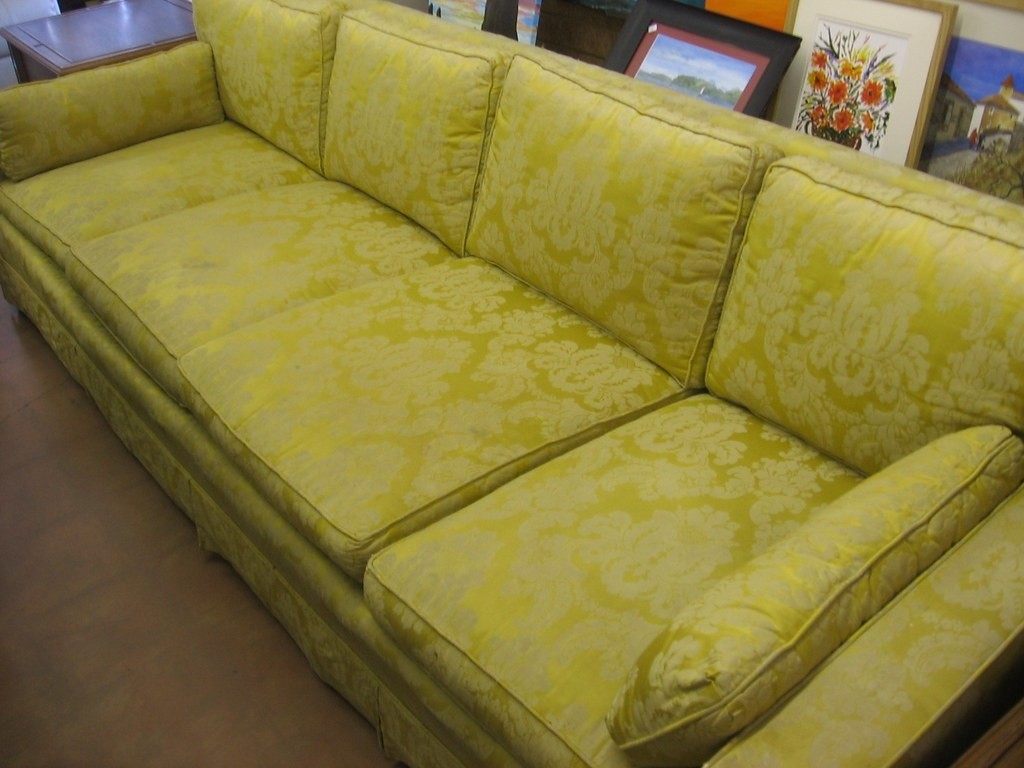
[766,0,1024,206]
[602,0,803,117]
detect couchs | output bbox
[0,0,1024,768]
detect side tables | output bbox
[0,0,197,84]
